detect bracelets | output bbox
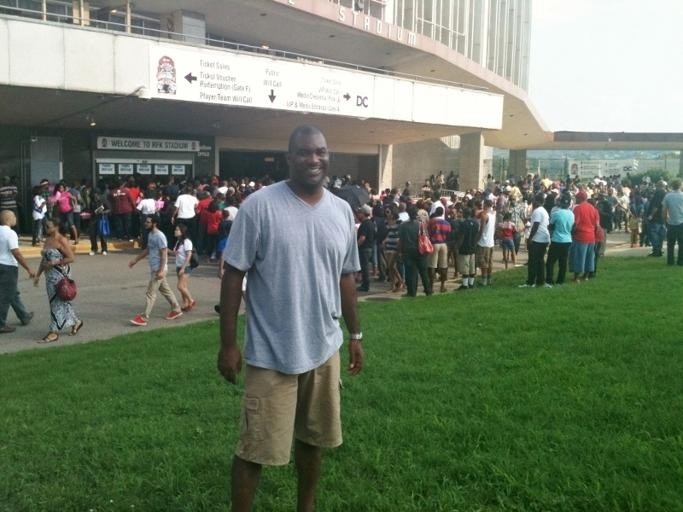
[347,330,364,343]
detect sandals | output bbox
[35,331,58,344]
[68,318,83,336]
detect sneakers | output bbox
[354,265,599,298]
[128,313,147,327]
[0,326,16,333]
[631,241,683,268]
[88,250,108,256]
[178,299,196,312]
[20,311,34,325]
[164,310,183,321]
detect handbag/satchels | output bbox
[180,238,199,270]
[54,274,78,301]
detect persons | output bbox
[214,123,367,511]
